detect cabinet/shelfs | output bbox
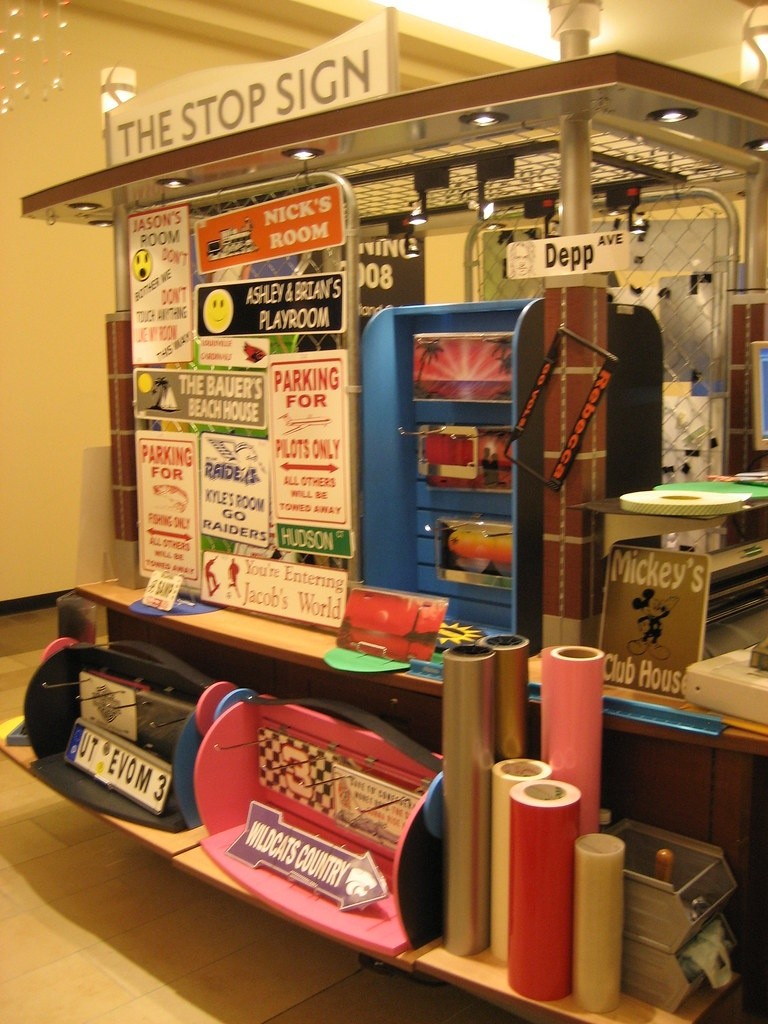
[0,52,768,1024]
